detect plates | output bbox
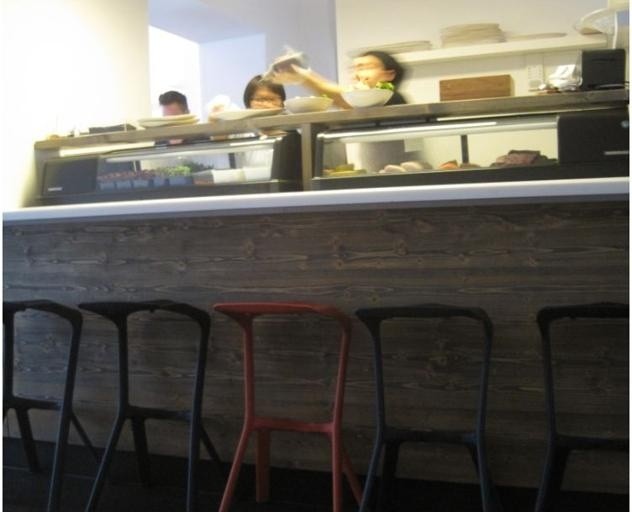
[208,109,284,121]
[283,96,333,113]
[135,114,202,128]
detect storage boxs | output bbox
[580,48,627,91]
[439,74,515,101]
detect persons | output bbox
[270,50,429,173]
[207,72,289,172]
[153,90,213,146]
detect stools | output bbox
[534,301,629,512]
[355,303,502,511]
[3,297,105,511]
[211,300,369,511]
[77,300,232,511]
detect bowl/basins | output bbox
[340,88,394,109]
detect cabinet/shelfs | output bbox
[307,86,629,192]
[32,111,307,207]
